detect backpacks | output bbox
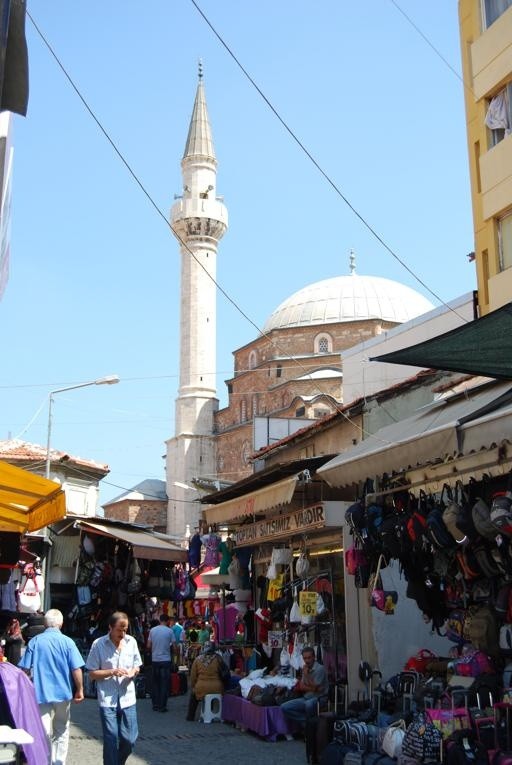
[345,473,510,652]
[68,534,196,645]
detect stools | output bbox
[198,692,222,724]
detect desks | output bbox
[221,692,288,743]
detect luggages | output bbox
[307,650,511,762]
[170,662,180,695]
[134,673,147,697]
[82,670,97,698]
[178,670,187,694]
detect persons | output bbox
[145,615,231,721]
[278,647,331,744]
[17,609,86,765]
[86,612,144,765]
[186,530,244,592]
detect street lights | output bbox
[38,373,120,612]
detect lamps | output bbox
[174,184,224,201]
